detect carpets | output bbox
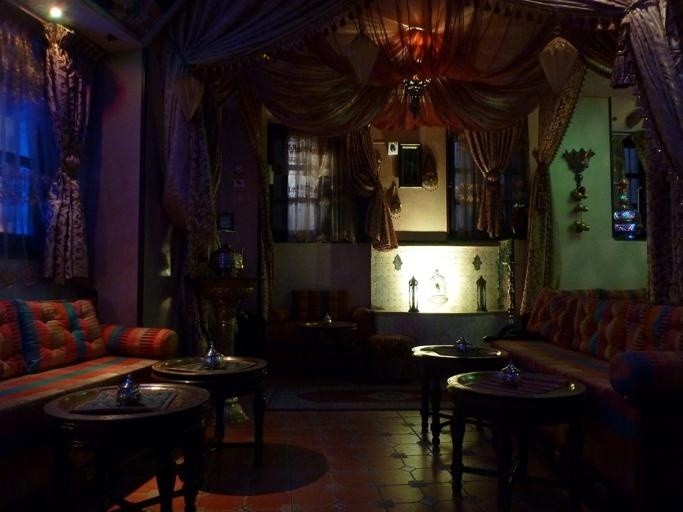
[263,372,455,410]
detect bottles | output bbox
[203,344,224,368]
[455,335,472,354]
[500,359,523,389]
[118,373,143,402]
[323,313,331,324]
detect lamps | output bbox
[401,26,433,119]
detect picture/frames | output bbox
[397,142,423,190]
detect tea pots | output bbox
[214,230,245,278]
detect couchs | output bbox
[484,286,683,491]
[2,298,178,465]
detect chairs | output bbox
[271,286,372,369]
